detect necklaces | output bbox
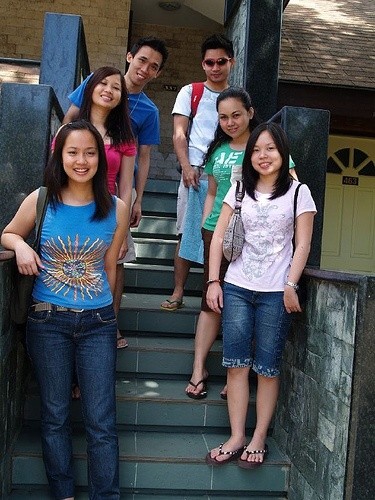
[127,92,142,116]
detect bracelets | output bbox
[205,278,220,285]
[285,281,299,292]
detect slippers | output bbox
[237,444,269,469]
[116,336,128,349]
[186,375,208,400]
[160,299,185,310]
[207,441,247,465]
[71,383,80,399]
[220,392,227,400]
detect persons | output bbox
[51,32,299,401]
[206,123,317,468]
[0,120,129,500]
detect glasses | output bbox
[203,57,232,66]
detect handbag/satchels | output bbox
[10,186,51,326]
[176,82,205,173]
[221,179,246,262]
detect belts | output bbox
[34,303,85,312]
